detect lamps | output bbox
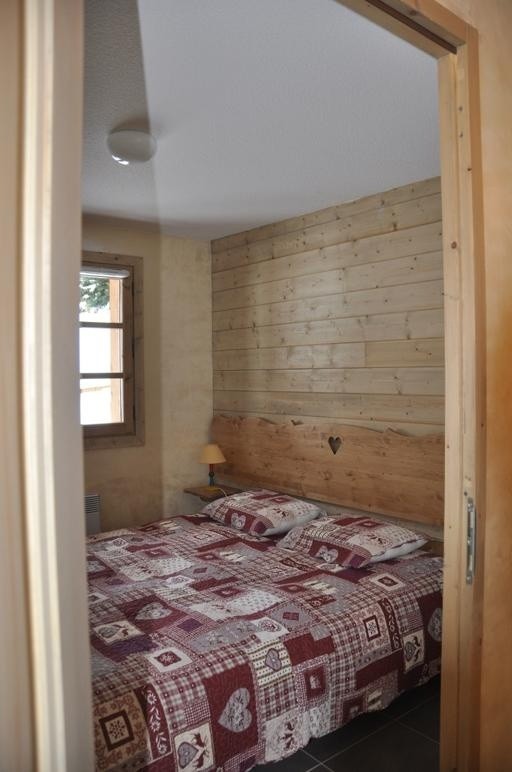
[197,442,227,492]
[106,124,158,167]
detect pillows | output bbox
[202,484,431,569]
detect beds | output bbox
[85,512,442,772]
